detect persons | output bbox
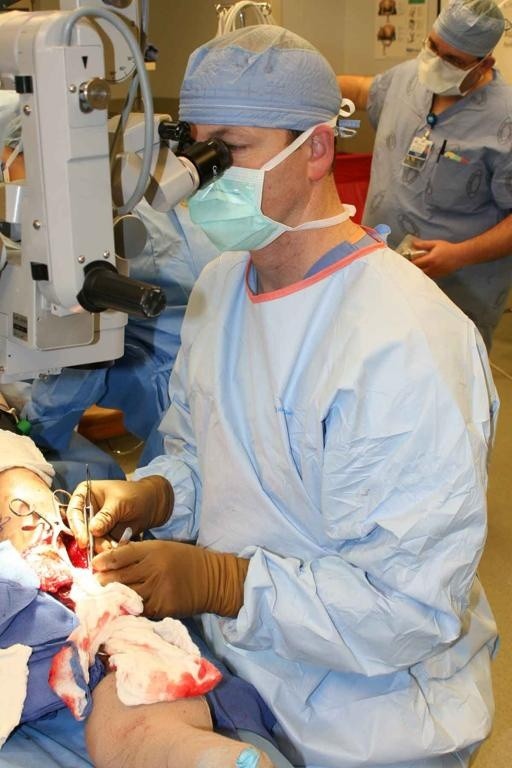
[314,3,512,356]
[0,87,222,463]
[65,21,502,768]
[2,390,279,768]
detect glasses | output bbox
[423,34,479,70]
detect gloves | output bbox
[66,476,174,554]
[93,540,250,620]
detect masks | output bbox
[188,165,288,252]
[417,46,469,96]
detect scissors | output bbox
[10,488,75,550]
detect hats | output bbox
[433,1,505,57]
[179,24,361,137]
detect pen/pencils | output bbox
[437,140,447,162]
